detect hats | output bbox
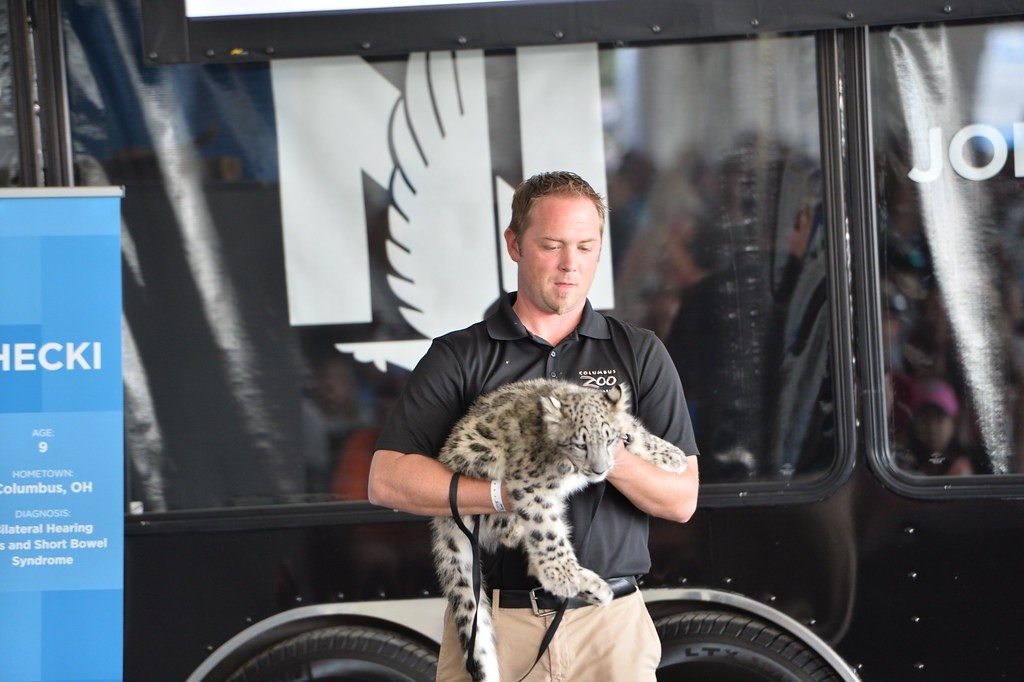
[910,377,958,418]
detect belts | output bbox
[486,575,637,618]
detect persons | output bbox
[899,379,968,481]
[304,142,826,504]
[365,171,701,682]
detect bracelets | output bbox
[490,479,507,515]
